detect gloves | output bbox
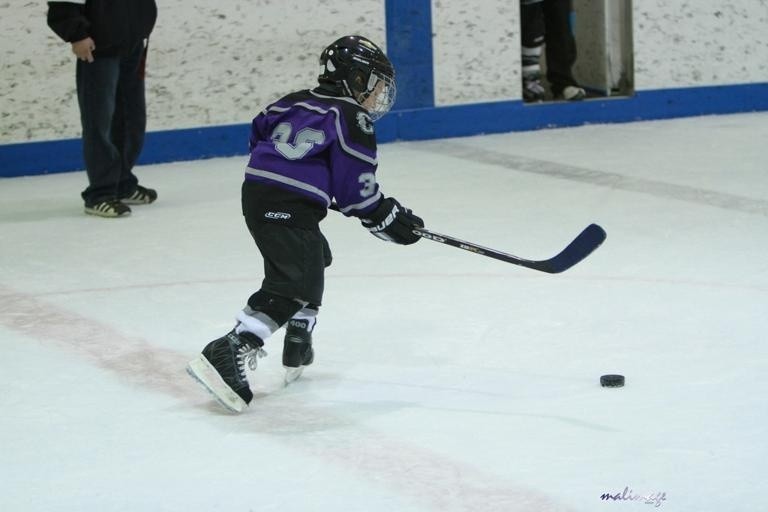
[360,197,423,244]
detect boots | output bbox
[198,320,267,404]
[282,318,316,367]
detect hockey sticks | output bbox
[329,203,606,273]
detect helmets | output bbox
[318,34,396,121]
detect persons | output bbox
[198,33,425,409]
[44,0,158,218]
[519,0,588,104]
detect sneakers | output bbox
[119,184,157,204]
[84,200,131,218]
[523,80,544,99]
[560,84,586,99]
